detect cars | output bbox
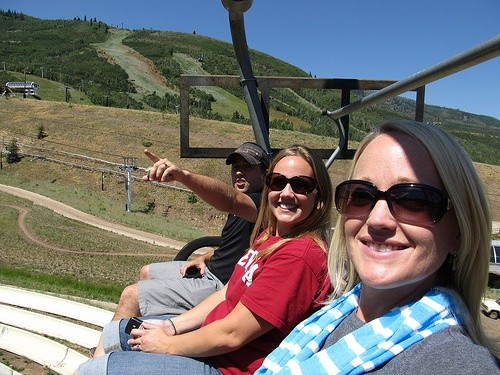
[481,295,500,321]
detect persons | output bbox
[253,118,500,375]
[73,146,333,375]
[109,141,270,321]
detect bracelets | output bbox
[169,319,177,336]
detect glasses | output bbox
[334,179,453,226]
[265,172,319,194]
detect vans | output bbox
[487,240,500,290]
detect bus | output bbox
[6,81,39,97]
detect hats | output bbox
[226,142,270,168]
